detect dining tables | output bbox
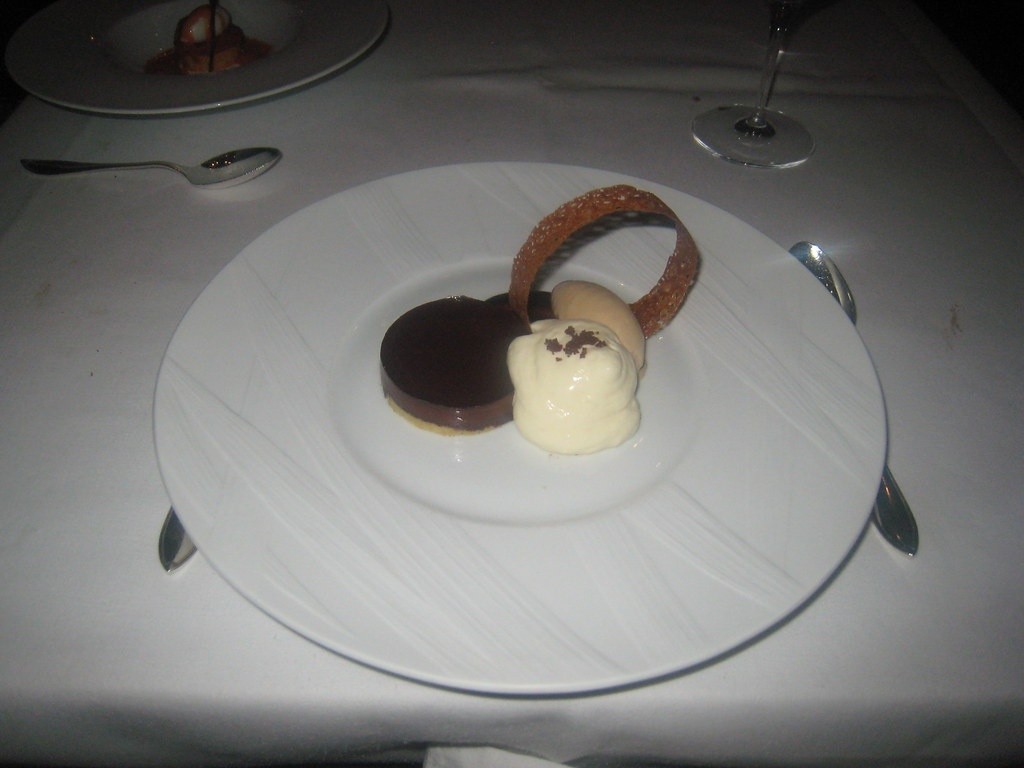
[0,2,1022,766]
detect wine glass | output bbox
[685,1,819,168]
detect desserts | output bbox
[172,0,249,76]
[379,183,700,454]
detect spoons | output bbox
[17,145,282,189]
[788,239,920,557]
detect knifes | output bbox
[160,495,196,570]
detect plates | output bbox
[1,2,388,114]
[152,161,883,693]
